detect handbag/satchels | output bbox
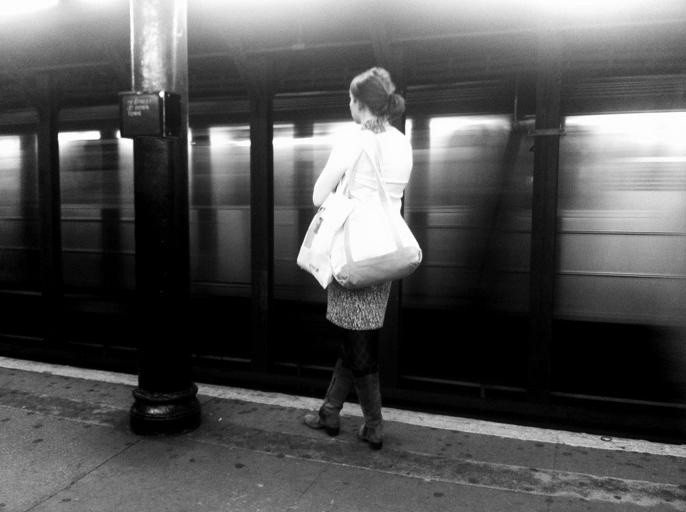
[330,199,422,289]
[296,190,355,290]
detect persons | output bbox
[303,65,415,453]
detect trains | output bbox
[0,110,686,328]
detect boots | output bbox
[304,355,351,436]
[354,371,385,451]
